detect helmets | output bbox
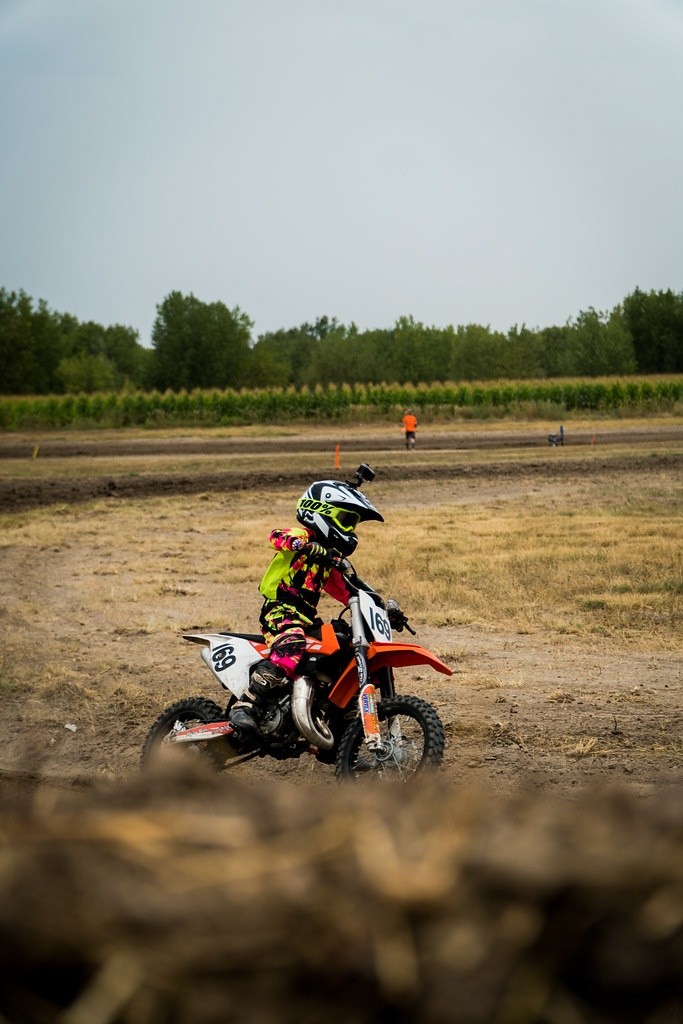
[297,478,384,555]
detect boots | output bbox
[229,659,285,731]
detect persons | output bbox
[401,408,418,451]
[228,479,408,732]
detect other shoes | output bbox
[405,442,415,451]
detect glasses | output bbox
[333,507,360,533]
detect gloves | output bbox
[303,541,327,562]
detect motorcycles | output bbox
[139,542,454,792]
